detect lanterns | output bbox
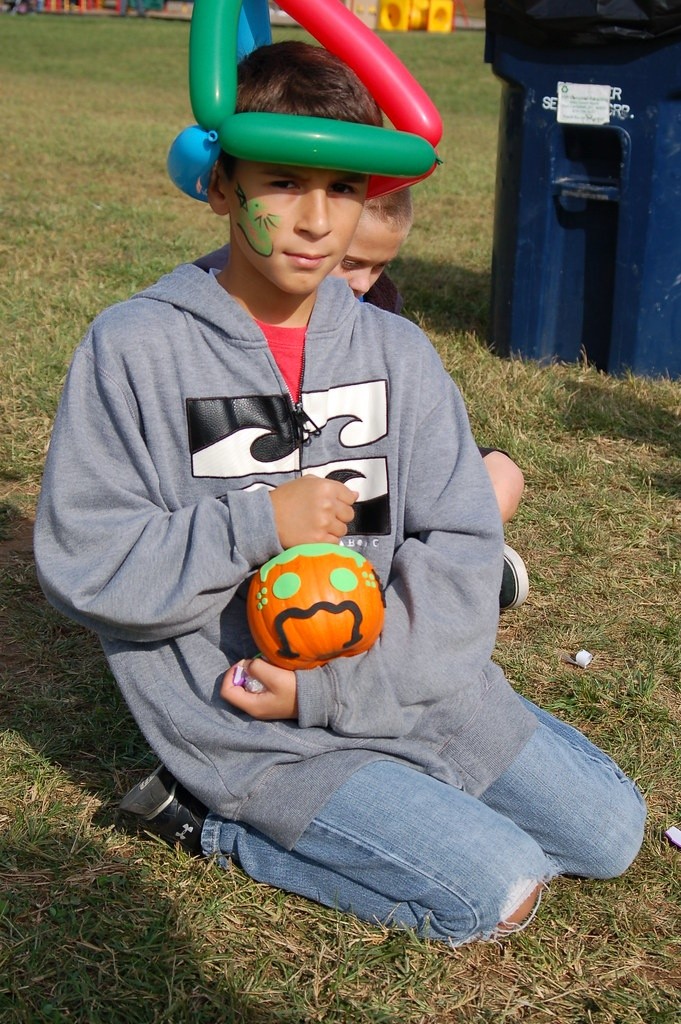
[246,546,382,670]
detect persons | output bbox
[32,41,646,942]
[187,182,531,616]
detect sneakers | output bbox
[118,762,208,861]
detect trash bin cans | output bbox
[483,0,681,382]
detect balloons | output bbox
[165,0,446,210]
